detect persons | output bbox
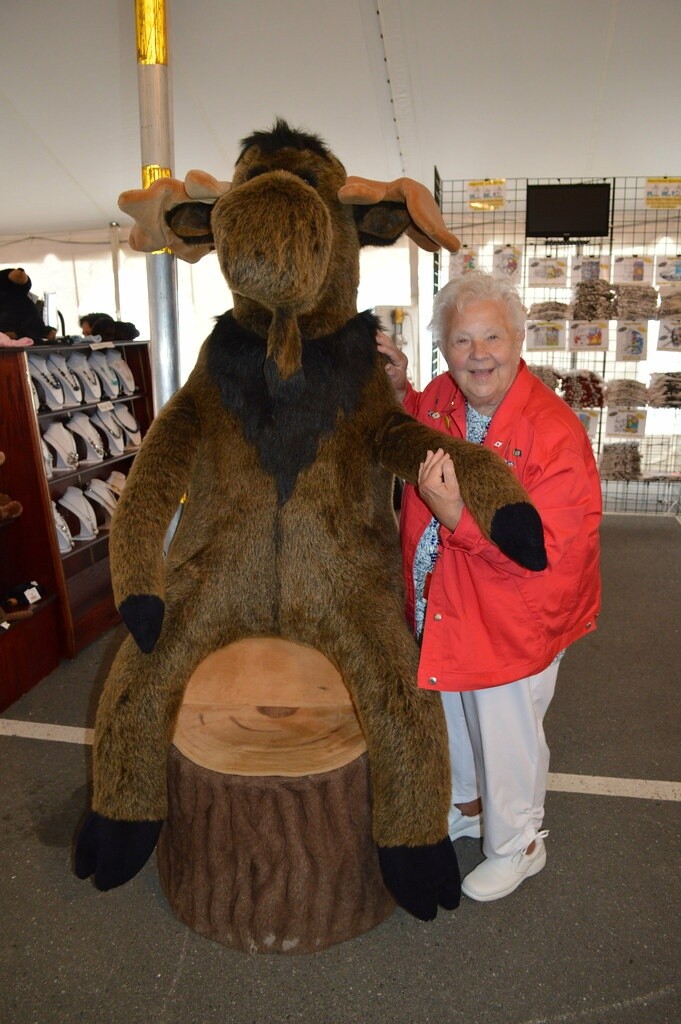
[371,269,603,900]
[78,312,114,338]
[27,349,142,553]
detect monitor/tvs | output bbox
[525,183,610,245]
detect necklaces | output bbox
[48,357,80,392]
[86,485,117,511]
[82,367,97,386]
[62,495,99,535]
[71,419,104,454]
[106,481,123,493]
[53,510,75,547]
[92,357,118,385]
[44,430,79,466]
[28,359,61,390]
[113,359,131,381]
[112,408,140,434]
[95,411,122,439]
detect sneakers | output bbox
[447,804,484,842]
[461,829,550,901]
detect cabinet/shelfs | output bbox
[0,338,156,714]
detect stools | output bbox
[157,704,396,952]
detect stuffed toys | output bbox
[0,452,23,520]
[0,580,39,622]
[71,116,546,923]
[0,268,56,347]
